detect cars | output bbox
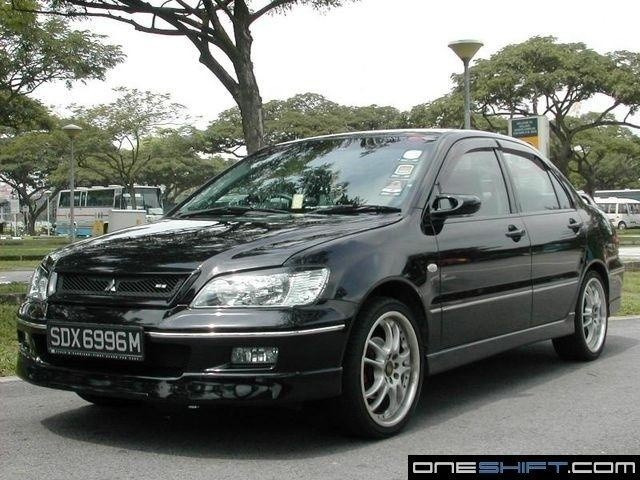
[16,127,625,442]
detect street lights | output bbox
[61,124,83,244]
[447,40,484,129]
[44,190,52,236]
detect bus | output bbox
[575,197,640,230]
[55,184,164,236]
